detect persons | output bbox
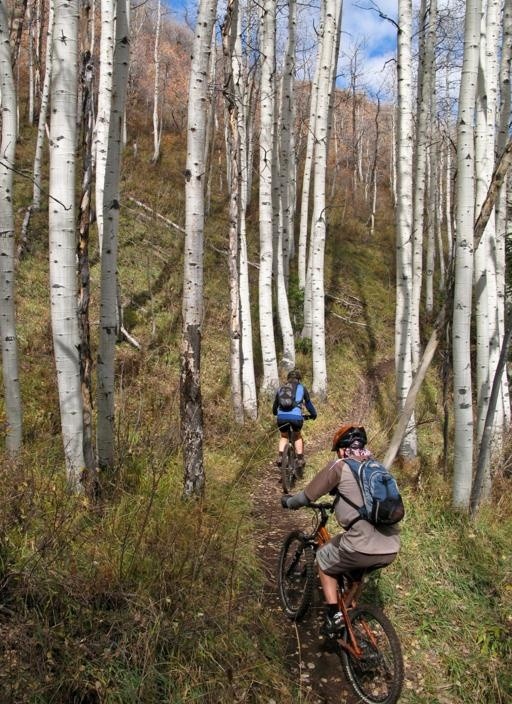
[280,424,405,661]
[272,370,317,467]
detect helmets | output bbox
[286,369,303,380]
[329,422,366,451]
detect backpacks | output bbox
[277,381,299,411]
[339,455,407,529]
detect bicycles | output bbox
[275,499,405,704]
[278,414,315,494]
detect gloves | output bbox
[280,494,292,507]
[309,414,317,420]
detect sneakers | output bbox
[296,459,306,468]
[318,611,346,637]
[275,455,282,464]
